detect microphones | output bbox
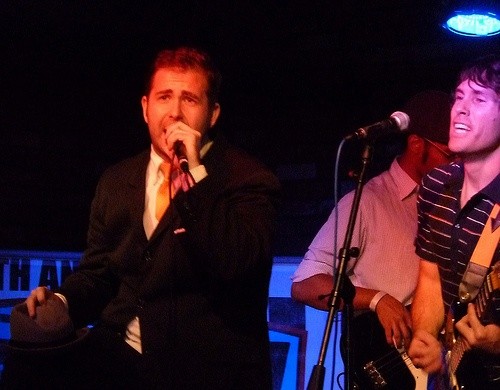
[347,111,410,141]
[173,140,189,174]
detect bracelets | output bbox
[369,291,387,311]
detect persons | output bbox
[0,46,281,390]
[408,57,500,390]
[290,90,464,355]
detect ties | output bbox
[154,160,176,221]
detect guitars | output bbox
[339,262,500,390]
